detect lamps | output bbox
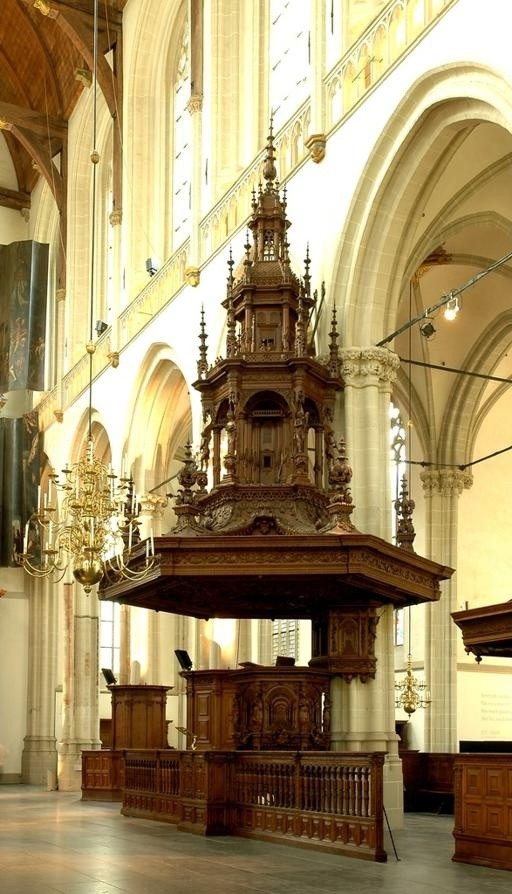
[20,1,157,591]
[393,280,431,723]
